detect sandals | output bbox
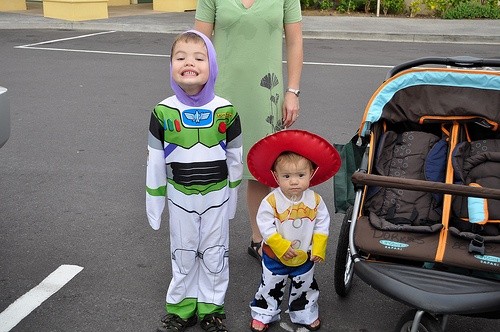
[250,319,269,332]
[303,319,321,331]
[247,241,263,263]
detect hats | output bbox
[247,128,342,187]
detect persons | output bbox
[246,129,342,332]
[145,31,243,332]
[194,0,303,265]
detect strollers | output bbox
[329,54,500,332]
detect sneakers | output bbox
[199,313,229,332]
[156,312,198,332]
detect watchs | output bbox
[286,89,301,96]
